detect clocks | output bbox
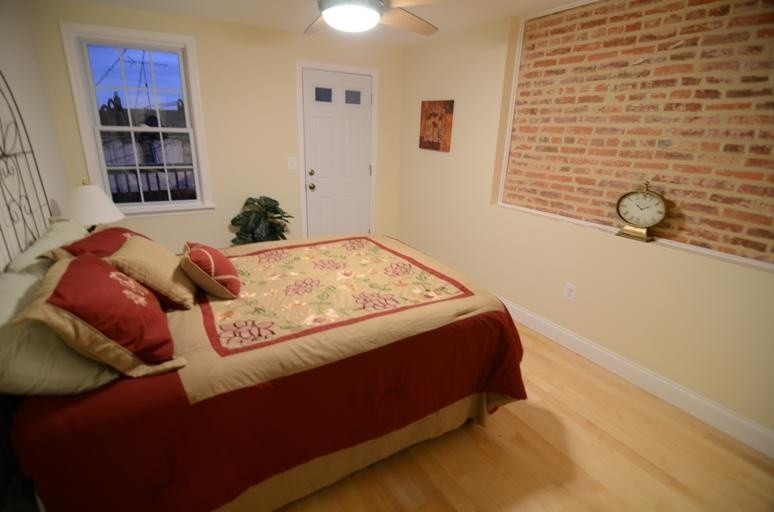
[613,182,669,242]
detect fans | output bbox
[303,1,440,36]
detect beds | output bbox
[0,72,528,512]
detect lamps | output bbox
[69,177,126,234]
[316,0,387,34]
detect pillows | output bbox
[37,227,197,310]
[0,272,120,393]
[5,221,90,274]
[179,242,241,299]
[8,256,187,378]
